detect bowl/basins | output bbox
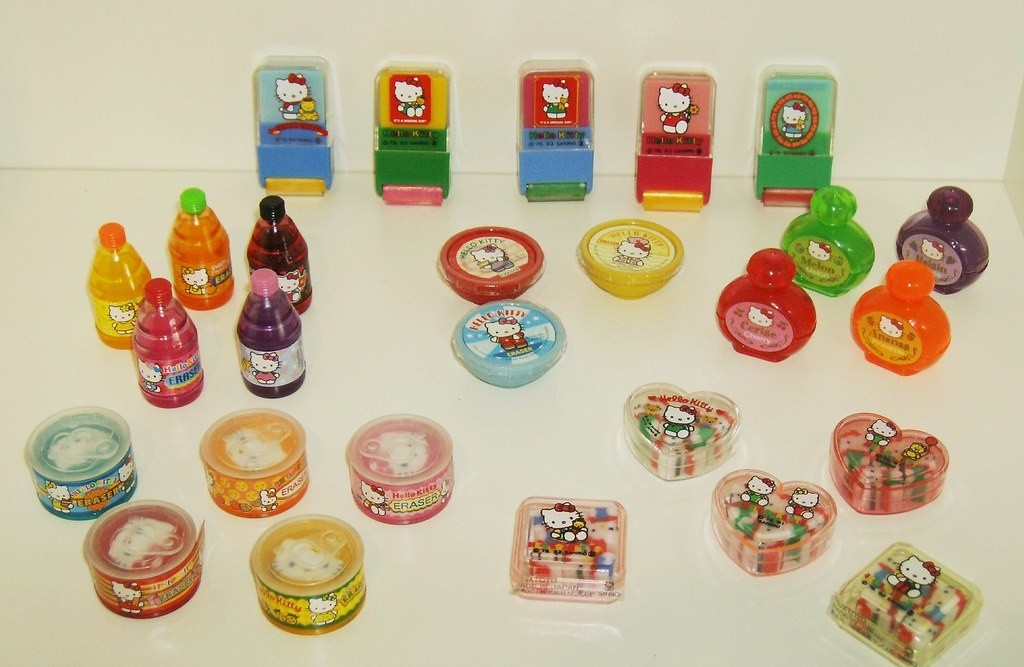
[449,298,565,389]
[576,219,686,302]
[438,226,544,305]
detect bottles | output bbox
[167,188,235,310]
[893,188,991,296]
[235,270,307,398]
[780,185,876,297]
[131,279,205,410]
[849,259,951,377]
[86,223,150,350]
[715,248,816,364]
[246,194,314,315]
